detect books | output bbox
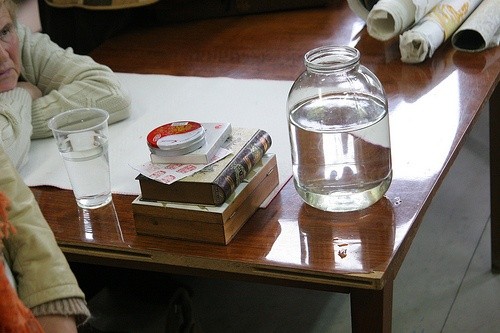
[131,123,272,205]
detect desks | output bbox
[27,0,500,332]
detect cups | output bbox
[48,107,113,209]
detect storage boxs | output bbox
[131,152,279,245]
[150,122,231,163]
[146,120,206,155]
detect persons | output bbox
[0,145,92,332]
[0,0,131,173]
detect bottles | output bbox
[285,43,393,212]
